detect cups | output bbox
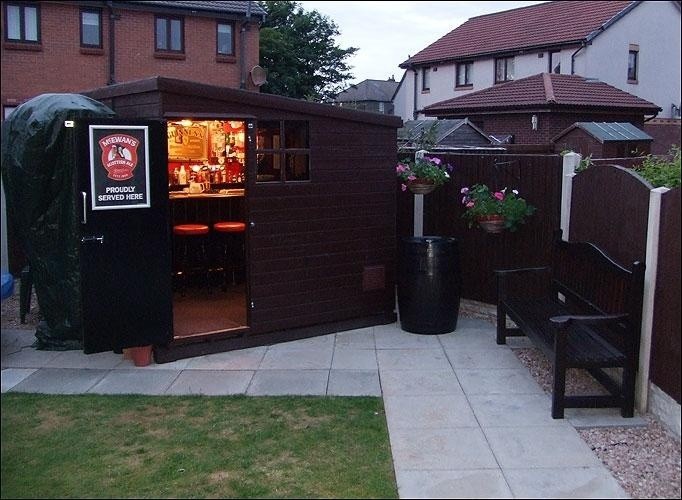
[190,183,205,193]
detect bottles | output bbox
[169,129,245,186]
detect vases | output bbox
[476,214,507,233]
[408,180,436,195]
[128,344,154,366]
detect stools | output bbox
[172,221,245,290]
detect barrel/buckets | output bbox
[397,236,461,335]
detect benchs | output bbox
[493,228,647,420]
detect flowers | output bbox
[396,155,454,191]
[460,183,538,229]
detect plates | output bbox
[169,191,190,196]
[220,189,245,195]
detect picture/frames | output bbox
[88,124,152,209]
